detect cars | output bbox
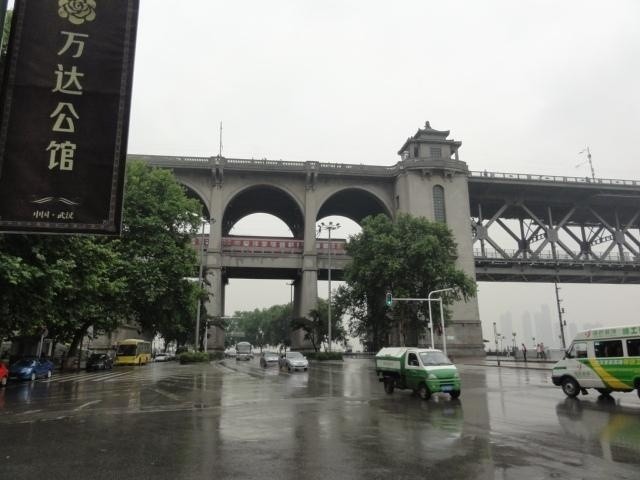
[11,358,57,382]
[278,352,309,373]
[155,353,177,363]
[259,352,280,369]
[87,353,115,370]
[0,363,9,387]
[226,342,256,362]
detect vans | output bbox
[549,324,640,402]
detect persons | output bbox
[540,342,547,360]
[521,343,527,362]
[536,344,541,359]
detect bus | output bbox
[111,338,153,367]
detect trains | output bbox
[187,234,349,256]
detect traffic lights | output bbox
[386,292,392,305]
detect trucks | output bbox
[375,346,462,401]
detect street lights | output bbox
[491,322,538,359]
[316,220,343,353]
[427,285,456,351]
[194,215,218,353]
